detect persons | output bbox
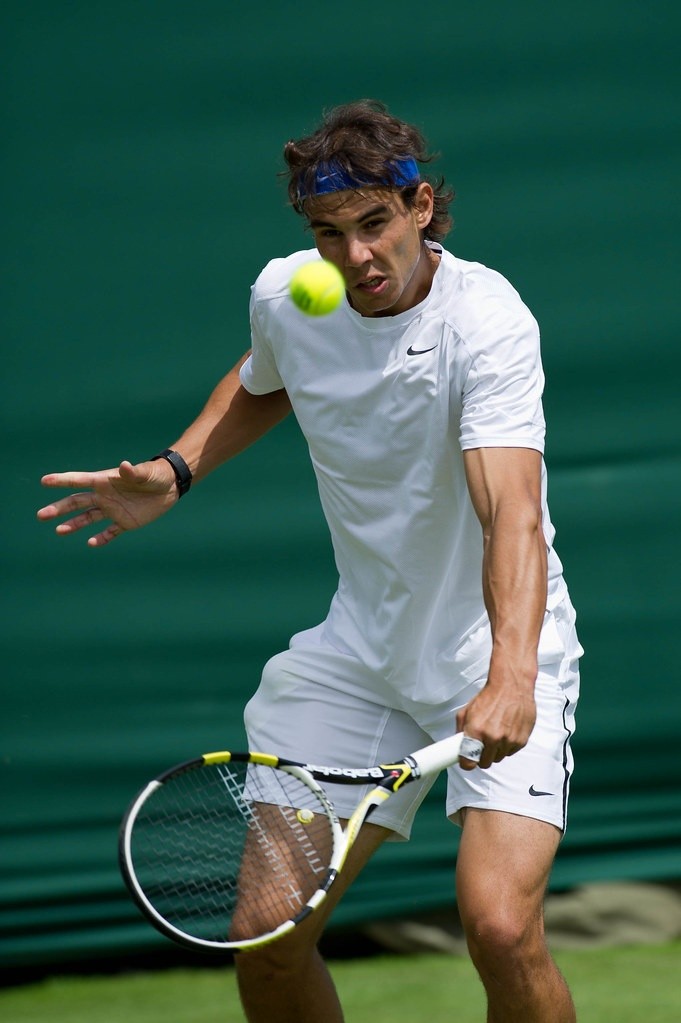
[38,101,583,1023]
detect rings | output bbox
[459,737,485,763]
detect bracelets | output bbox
[151,449,192,499]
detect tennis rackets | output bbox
[117,731,469,955]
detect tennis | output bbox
[290,260,345,316]
